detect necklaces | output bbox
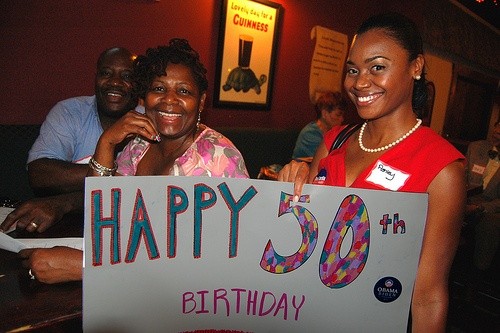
[358,118,422,153]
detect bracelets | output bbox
[89,158,119,177]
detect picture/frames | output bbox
[212,0,282,112]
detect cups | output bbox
[238,35,254,69]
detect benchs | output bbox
[0,123,49,203]
[213,126,302,179]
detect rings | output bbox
[30,221,39,228]
[29,268,36,280]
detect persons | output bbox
[0,37,500,284]
[278,10,468,333]
